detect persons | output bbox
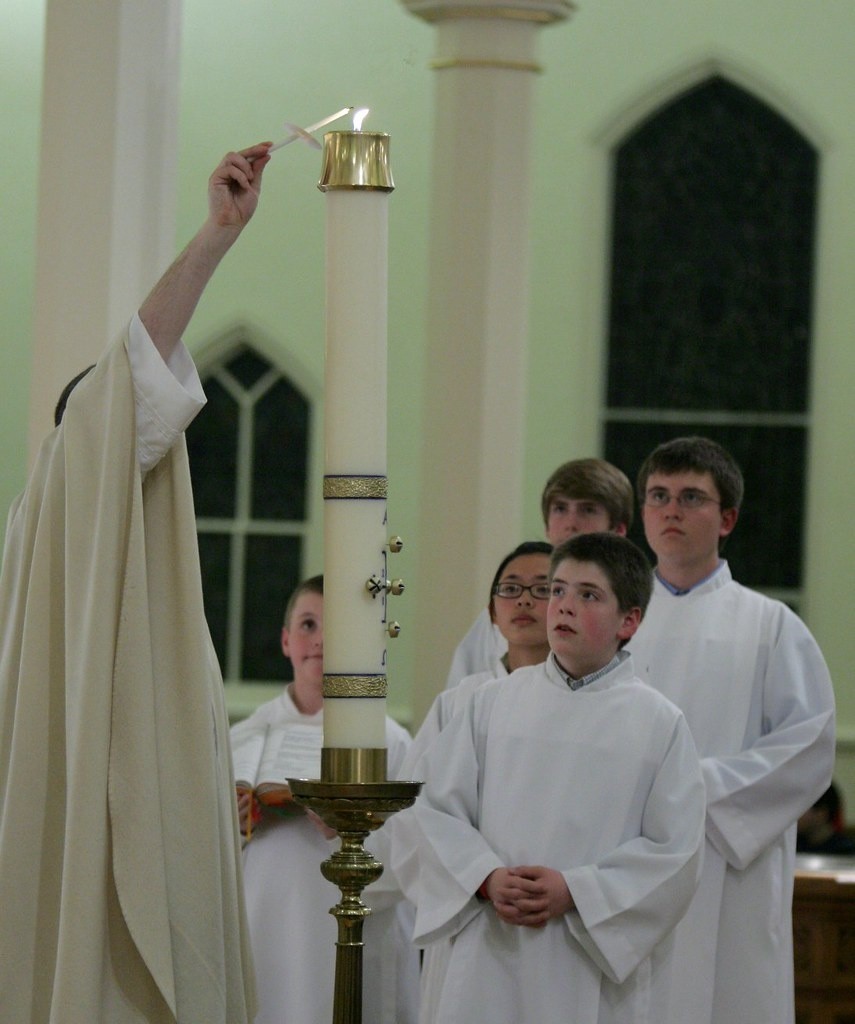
[0,141,271,1024]
[388,437,855,1024]
[229,574,414,1024]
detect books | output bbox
[233,780,307,829]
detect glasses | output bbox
[644,491,719,507]
[493,582,550,600]
